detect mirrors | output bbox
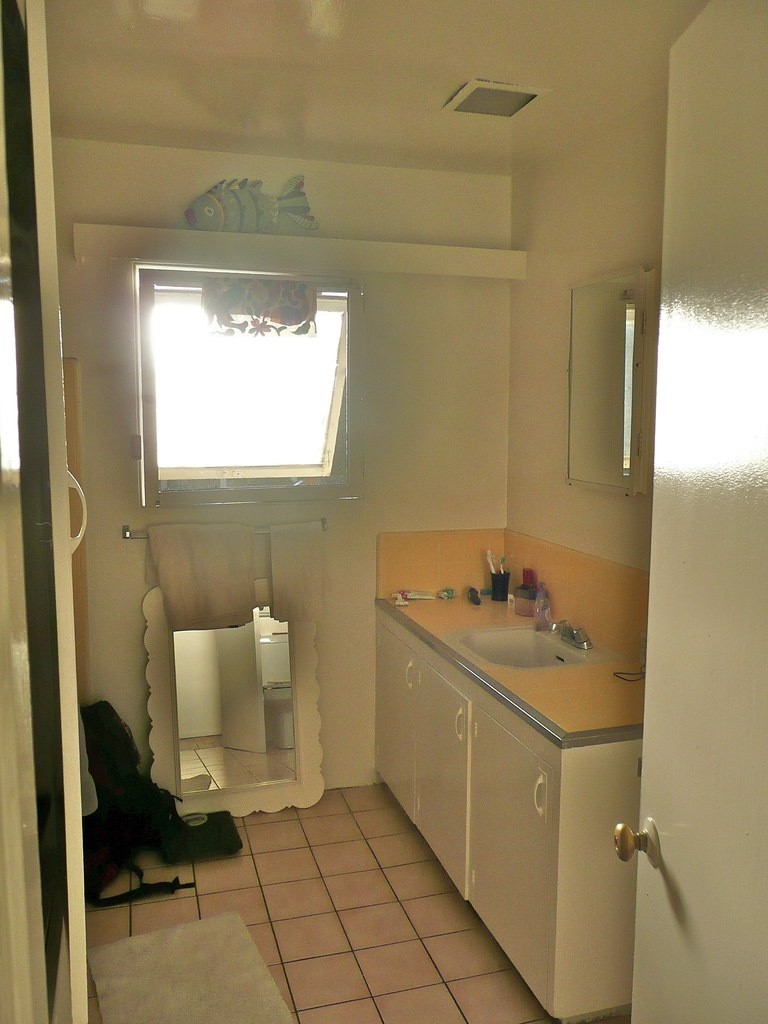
[566,265,644,499]
[141,580,324,819]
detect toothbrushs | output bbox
[500,554,506,574]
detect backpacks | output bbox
[80,700,196,907]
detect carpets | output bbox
[88,911,295,1024]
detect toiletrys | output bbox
[391,590,436,600]
[487,549,497,574]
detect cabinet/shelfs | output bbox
[375,604,644,1024]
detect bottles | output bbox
[534,582,552,630]
[513,568,536,615]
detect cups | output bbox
[489,571,511,600]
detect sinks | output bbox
[458,625,589,669]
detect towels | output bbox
[145,521,256,631]
[269,520,329,623]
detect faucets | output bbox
[550,619,594,650]
